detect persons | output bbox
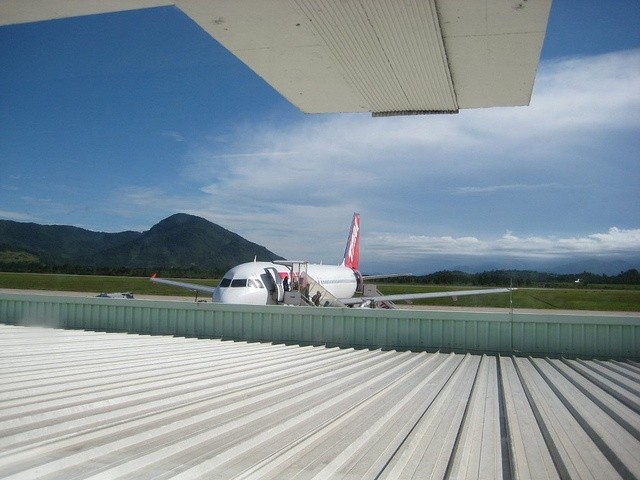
[312,291,321,306]
[296,277,304,292]
[290,278,297,291]
[283,276,290,291]
[302,282,310,303]
[368,298,378,308]
[324,301,329,307]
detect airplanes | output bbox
[149,213,519,308]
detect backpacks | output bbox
[312,295,317,302]
